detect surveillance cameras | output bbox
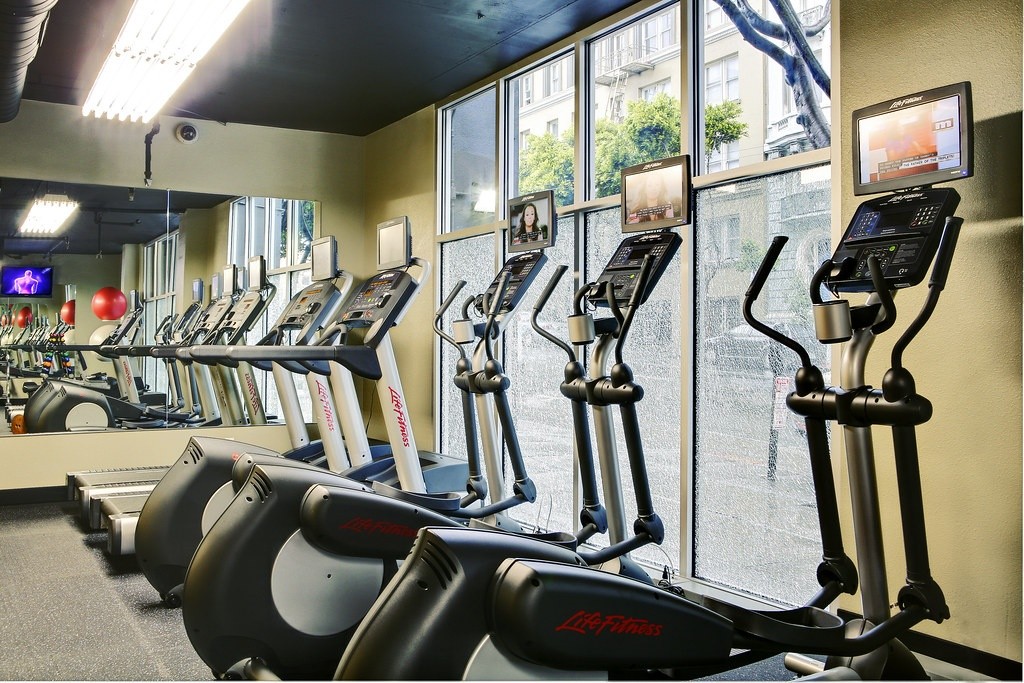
[176,124,199,144]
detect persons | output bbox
[626,173,674,224]
[14,270,38,294]
[512,203,543,244]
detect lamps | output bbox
[19,181,77,234]
[81,0,252,125]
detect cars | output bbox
[705,321,830,376]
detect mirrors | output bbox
[0,176,322,438]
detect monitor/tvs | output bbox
[311,235,338,281]
[129,290,138,313]
[247,255,266,290]
[507,189,558,253]
[191,264,238,302]
[621,154,692,233]
[377,216,412,271]
[2,266,54,299]
[851,81,975,196]
